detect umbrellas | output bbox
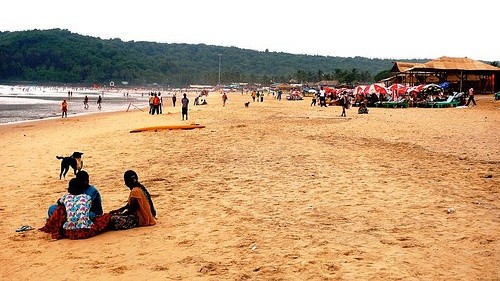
[408,55,500,93]
[353,83,443,102]
[322,85,355,100]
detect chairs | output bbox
[288,92,303,99]
[428,92,461,108]
[356,98,406,108]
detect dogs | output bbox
[55,151,85,180]
[244,102,250,108]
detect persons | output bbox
[181,93,189,120]
[339,94,347,117]
[148,91,163,115]
[172,95,176,107]
[193,90,207,106]
[465,88,477,107]
[97,96,102,108]
[252,91,264,102]
[84,95,88,108]
[61,99,68,118]
[318,87,328,107]
[222,93,227,107]
[310,92,318,106]
[275,89,282,101]
[38,169,157,240]
